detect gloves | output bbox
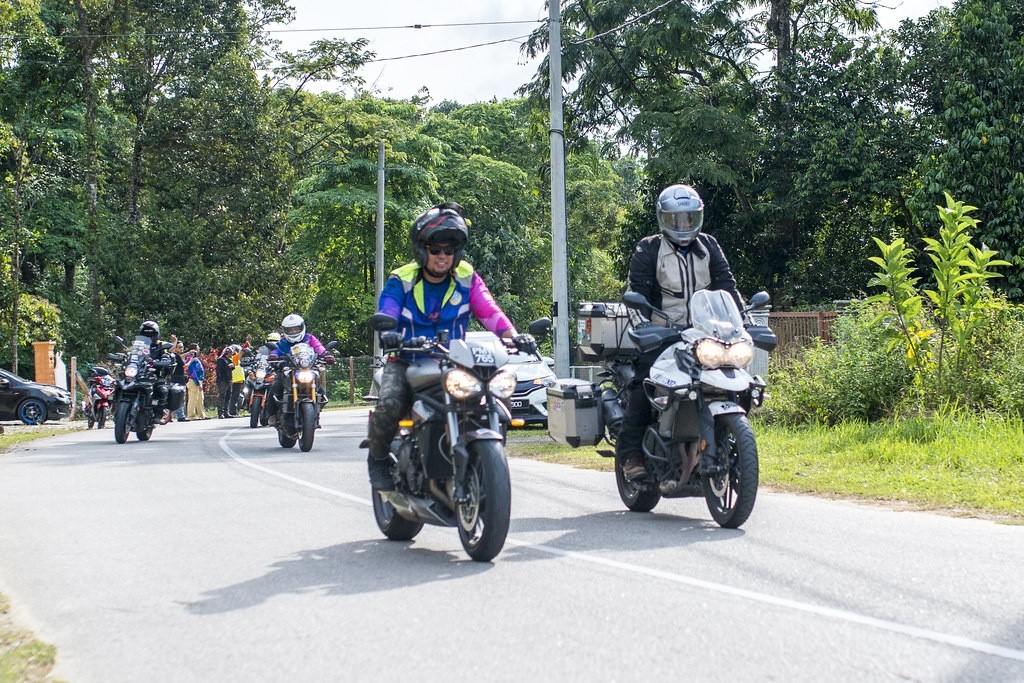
[381,331,400,350]
[325,355,335,365]
[513,334,537,355]
[268,354,278,360]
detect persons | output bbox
[154,333,196,421]
[266,314,335,428]
[186,343,211,419]
[238,331,282,425]
[615,184,747,484]
[229,334,252,415]
[112,320,170,425]
[216,347,235,419]
[369,201,536,490]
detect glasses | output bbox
[670,214,694,224]
[425,243,458,256]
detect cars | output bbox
[0,368,73,425]
[465,331,557,430]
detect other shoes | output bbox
[268,415,276,426]
[167,410,240,422]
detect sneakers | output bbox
[153,409,164,424]
[623,453,648,480]
[368,453,393,489]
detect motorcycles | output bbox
[105,335,178,444]
[85,361,117,429]
[240,345,274,428]
[263,341,339,452]
[359,312,553,562]
[546,290,779,528]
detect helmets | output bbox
[267,332,281,343]
[656,184,704,246]
[140,320,160,340]
[410,202,468,267]
[282,314,305,342]
